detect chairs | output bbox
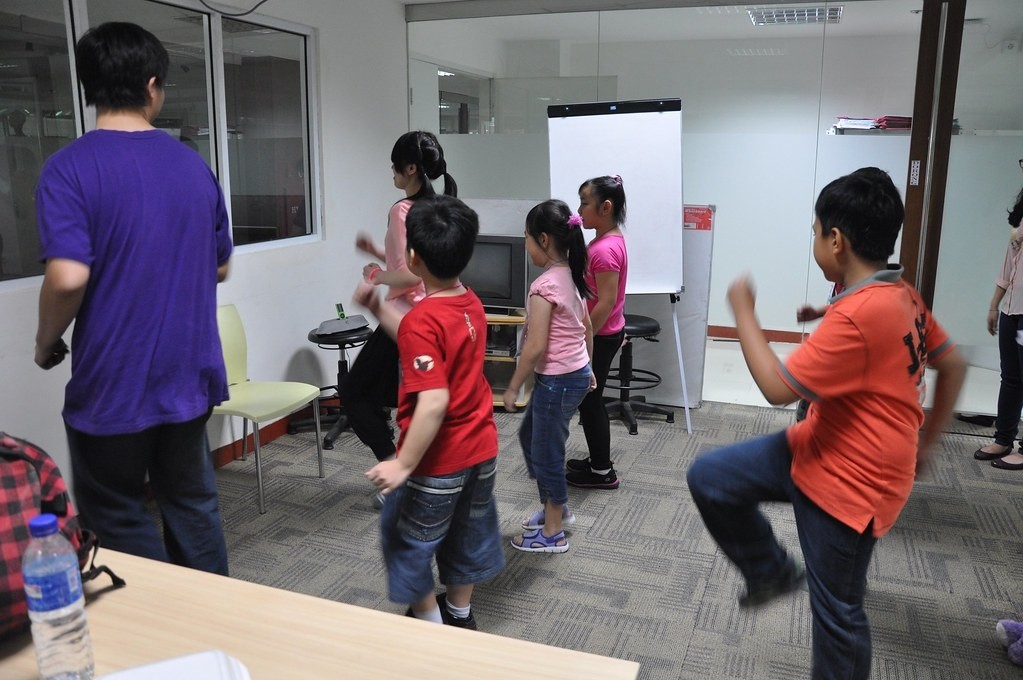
[201,307,332,512]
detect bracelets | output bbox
[369,267,382,285]
[989,310,999,311]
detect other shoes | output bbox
[405,605,415,618]
[991,458,1023,470]
[435,592,477,630]
[367,491,385,514]
[974,447,1012,460]
[738,555,805,610]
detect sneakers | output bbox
[566,467,619,489]
[566,457,614,472]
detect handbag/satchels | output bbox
[0,432,125,660]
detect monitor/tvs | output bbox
[458,234,529,316]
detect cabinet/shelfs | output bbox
[477,309,528,415]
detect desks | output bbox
[0,546,642,680]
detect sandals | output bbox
[510,529,569,553]
[521,506,576,528]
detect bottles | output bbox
[21,513,96,680]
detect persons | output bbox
[354,131,458,509]
[997,619,1023,666]
[505,200,597,553]
[365,195,505,629]
[568,175,627,491]
[34,21,231,580]
[974,153,1023,471]
[686,167,969,679]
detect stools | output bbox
[604,314,675,434]
[307,323,383,450]
